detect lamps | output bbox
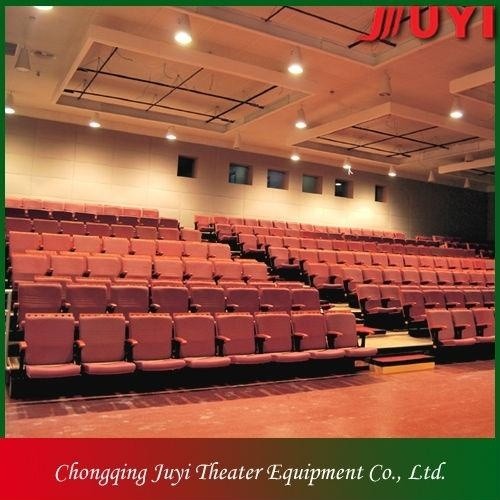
[287,44,306,77]
[4,92,17,115]
[172,13,193,47]
[295,108,310,131]
[13,45,34,74]
[447,94,465,121]
[378,73,392,98]
